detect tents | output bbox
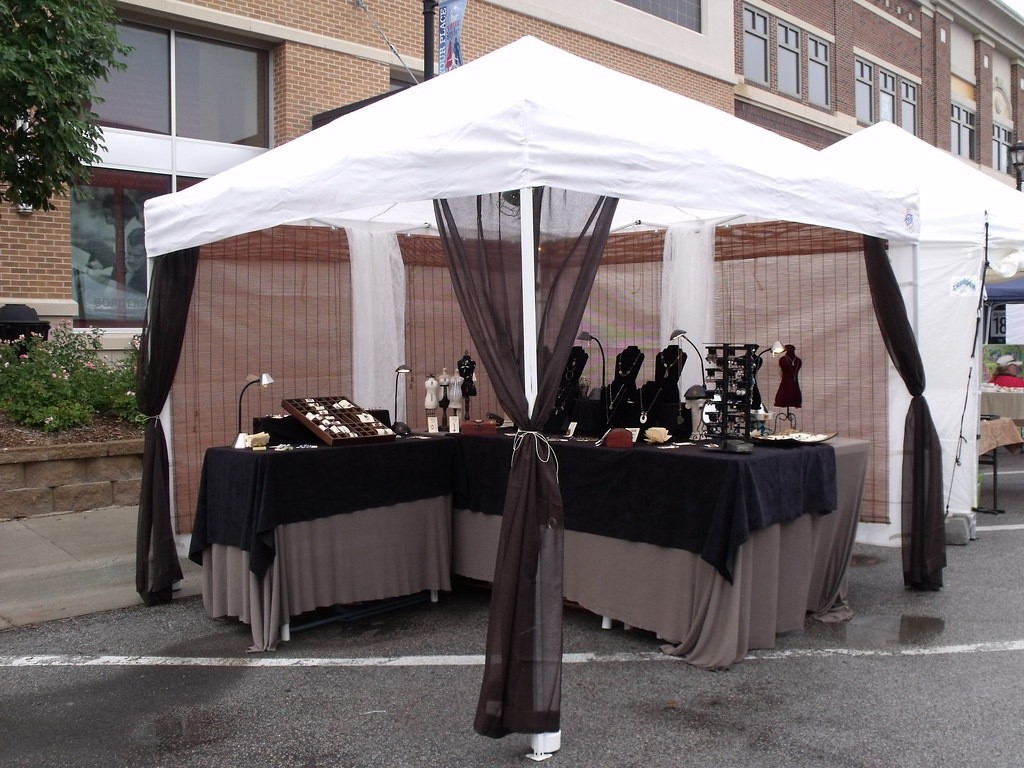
[136,32,947,761]
[819,118,1024,542]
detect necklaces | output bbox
[639,388,661,424]
[555,383,573,415]
[606,383,626,424]
[565,366,577,380]
[618,353,641,377]
[785,356,797,366]
[660,352,680,378]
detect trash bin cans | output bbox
[0,321,51,341]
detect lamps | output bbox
[391,365,413,435]
[576,328,607,400]
[230,373,275,449]
[669,327,705,400]
[758,341,785,413]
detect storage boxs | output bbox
[461,417,498,436]
[281,396,396,446]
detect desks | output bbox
[453,429,871,672]
[979,414,1024,513]
[190,434,453,655]
[978,384,1024,454]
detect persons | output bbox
[73,193,149,320]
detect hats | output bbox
[996,355,1023,368]
